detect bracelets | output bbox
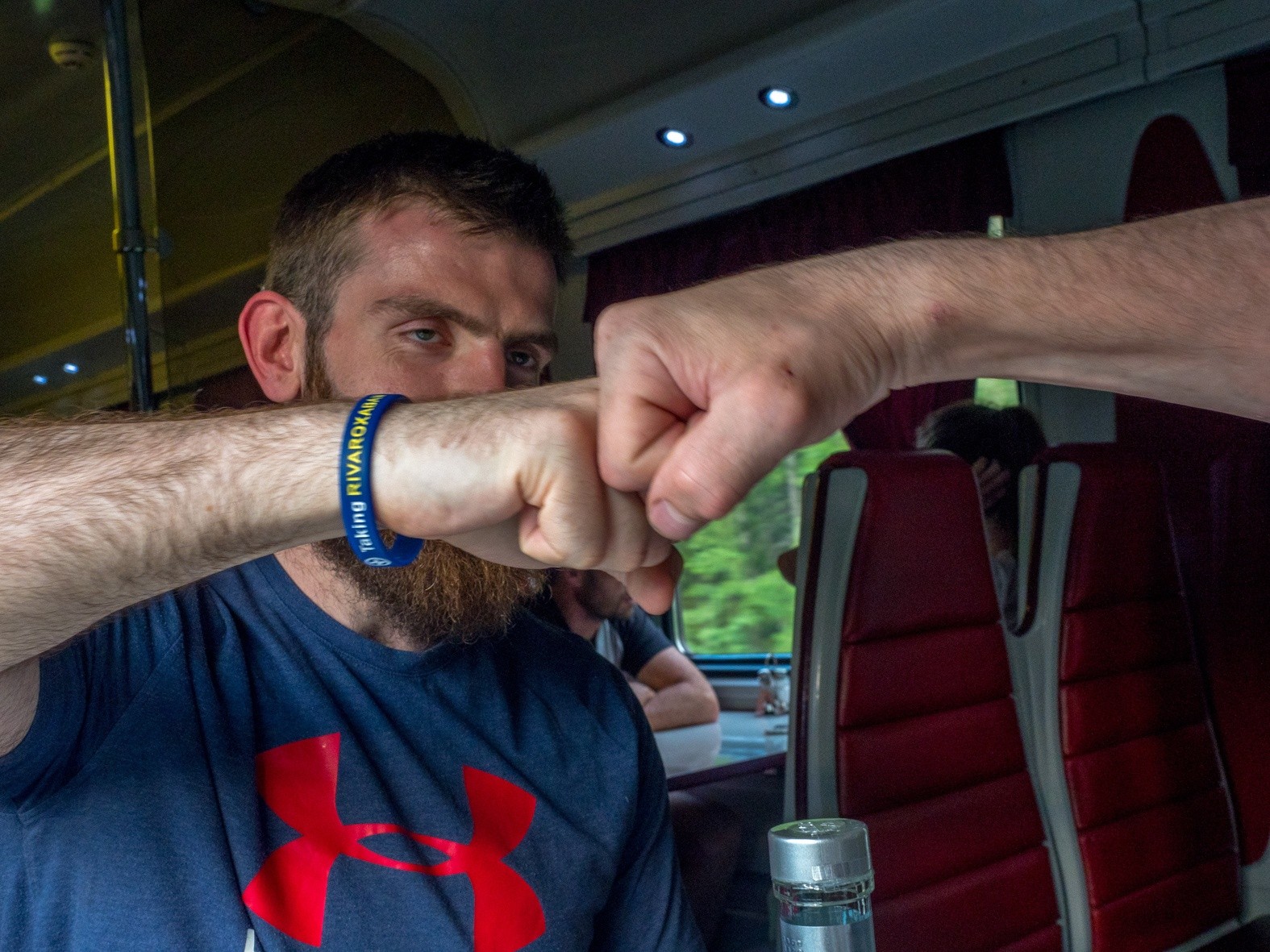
[338,393,426,568]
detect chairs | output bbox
[781,439,1243,952]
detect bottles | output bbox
[770,668,791,715]
[767,818,878,952]
[755,668,774,714]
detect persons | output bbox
[522,400,1051,952]
[0,130,703,952]
[591,195,1270,542]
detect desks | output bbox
[647,708,789,792]
